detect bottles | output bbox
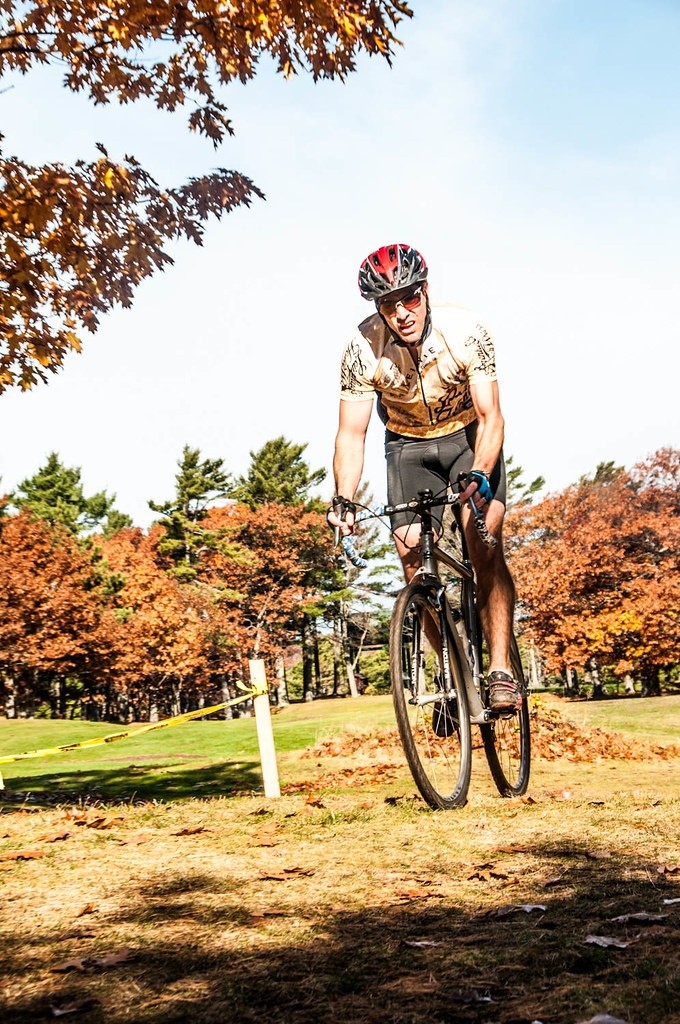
[451,610,473,658]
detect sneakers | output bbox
[487,671,521,710]
[432,675,460,737]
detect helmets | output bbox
[358,243,428,301]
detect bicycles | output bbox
[331,471,531,813]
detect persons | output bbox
[326,244,523,737]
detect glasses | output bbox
[376,286,423,319]
